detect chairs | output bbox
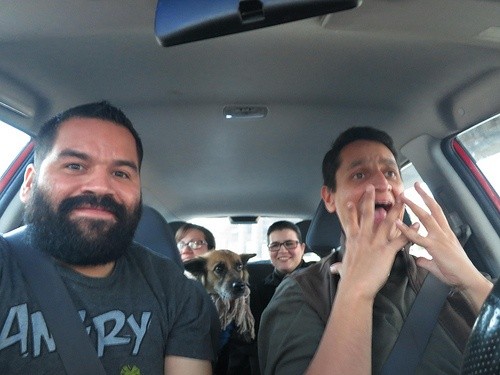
[305,198,419,262]
[3,204,184,270]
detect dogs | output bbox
[183,249,256,344]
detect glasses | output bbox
[268,241,302,251]
[177,240,209,252]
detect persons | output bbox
[0,101,221,374]
[174,223,249,375]
[224,220,320,374]
[257,126,500,375]
[206,260,207,261]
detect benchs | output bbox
[167,220,312,375]
